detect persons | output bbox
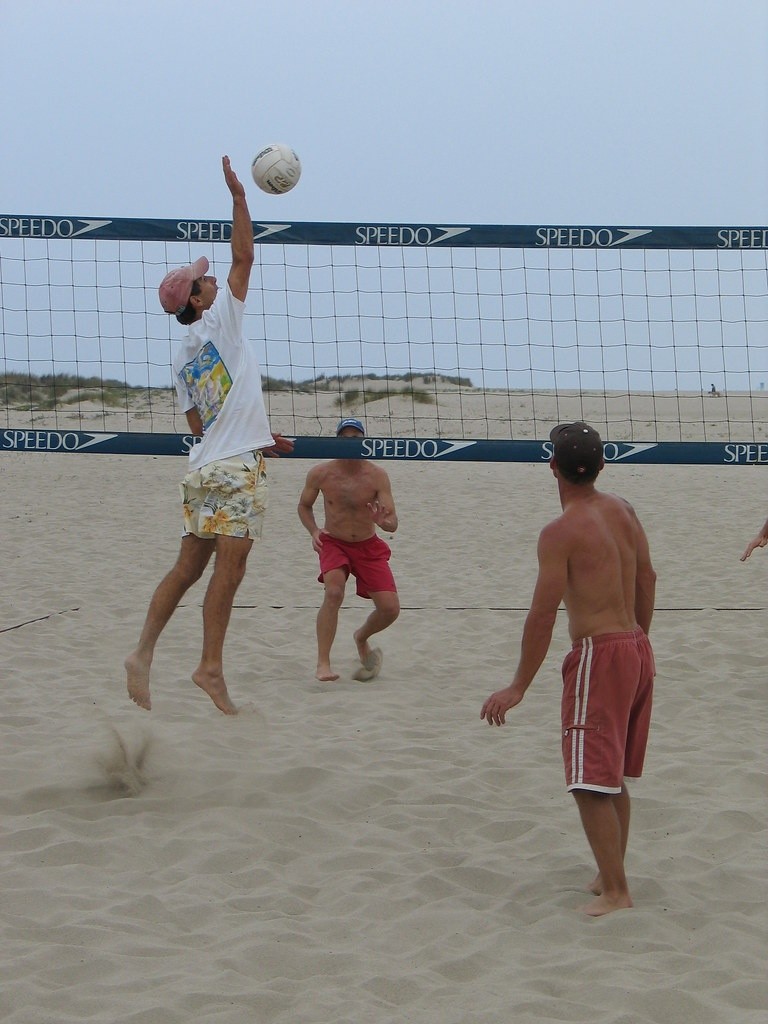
[479,419,658,917]
[711,384,715,392]
[124,153,293,714]
[298,418,399,682]
[740,517,768,562]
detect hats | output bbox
[159,256,209,315]
[551,421,603,480]
[337,419,364,437]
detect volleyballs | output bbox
[250,143,303,196]
